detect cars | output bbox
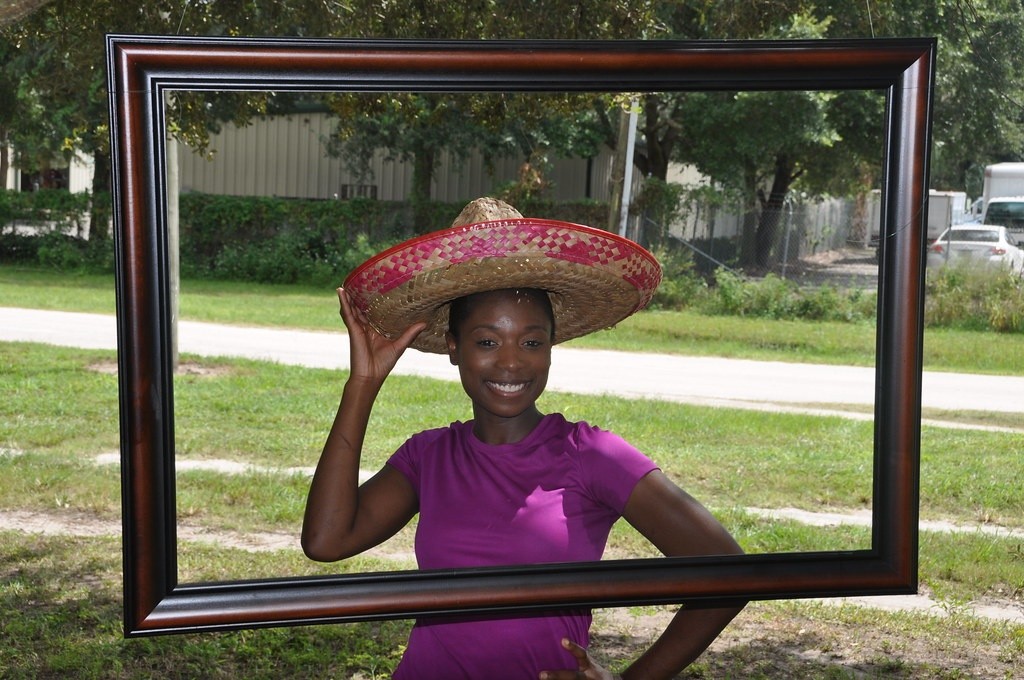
[931,224,1024,276]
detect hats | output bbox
[344,196,665,353]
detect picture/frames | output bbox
[103,32,940,639]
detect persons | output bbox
[301,198,751,680]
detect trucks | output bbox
[926,193,984,239]
[983,162,1024,246]
